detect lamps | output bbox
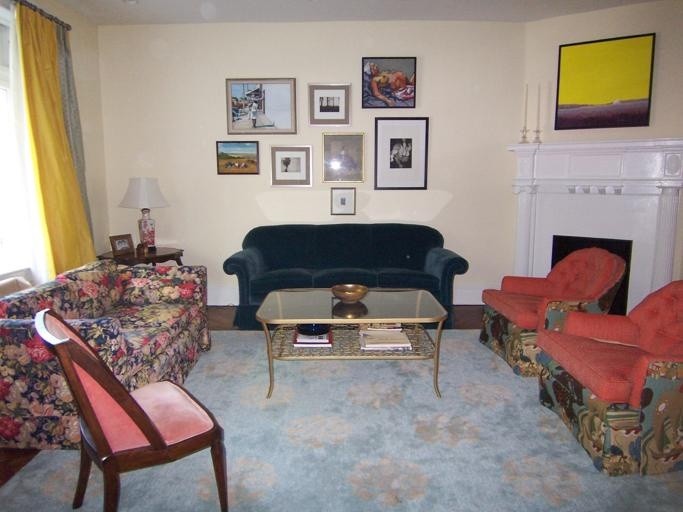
[116,177,170,246]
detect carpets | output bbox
[0,320,681,511]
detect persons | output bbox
[364,61,415,108]
[225,160,247,168]
[249,99,258,127]
[117,240,128,251]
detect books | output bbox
[293,331,332,347]
[359,329,413,352]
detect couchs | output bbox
[478,245,627,378]
[534,278,682,477]
[0,259,215,452]
[223,218,470,328]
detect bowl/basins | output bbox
[331,302,367,318]
[331,284,368,304]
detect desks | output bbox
[101,246,184,267]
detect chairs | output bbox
[32,308,229,511]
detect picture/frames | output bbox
[268,145,312,188]
[306,81,352,127]
[109,233,134,256]
[322,131,364,184]
[373,116,428,191]
[222,77,298,135]
[360,56,416,110]
[216,139,259,175]
[553,32,657,130]
[330,187,356,216]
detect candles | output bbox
[522,84,527,128]
[534,83,541,130]
